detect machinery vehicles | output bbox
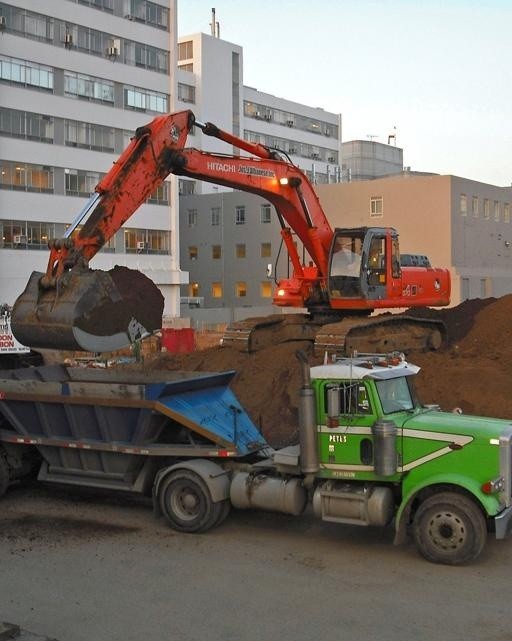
[9,108,452,360]
[2,348,511,566]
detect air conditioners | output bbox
[274,144,335,162]
[14,235,26,244]
[255,111,293,126]
[0,16,117,56]
[137,242,144,249]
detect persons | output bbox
[130,341,143,364]
[155,336,162,353]
